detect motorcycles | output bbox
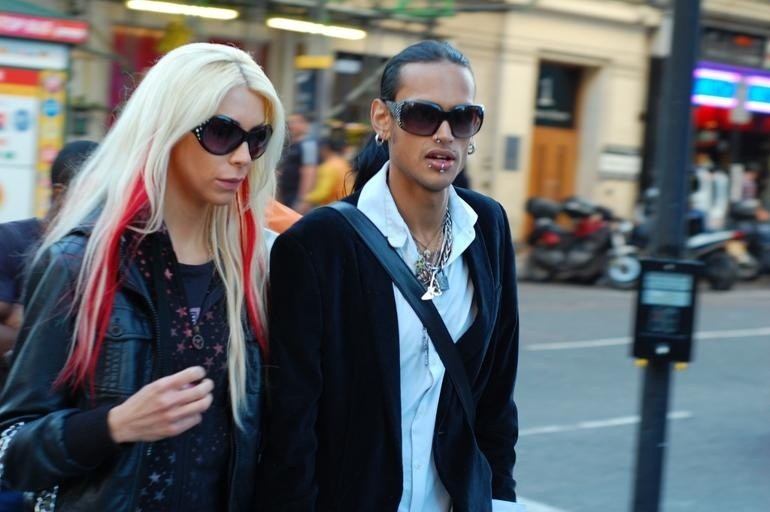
[525,189,770,289]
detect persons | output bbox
[0,43,282,511]
[274,105,319,215]
[742,163,763,200]
[258,40,522,512]
[306,137,357,206]
[0,140,101,358]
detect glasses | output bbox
[191,113,272,159]
[385,100,486,139]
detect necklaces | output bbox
[413,207,454,302]
